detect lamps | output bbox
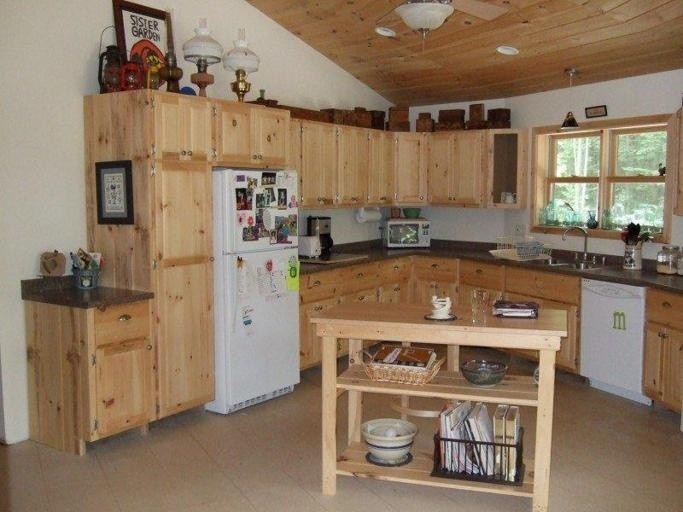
[223,28,261,103]
[561,67,580,129]
[184,20,224,97]
[395,0,455,40]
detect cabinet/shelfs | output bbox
[414,255,460,306]
[84,93,216,161]
[300,261,382,371]
[310,301,568,512]
[504,266,582,375]
[21,275,158,457]
[460,259,507,306]
[291,118,532,209]
[641,288,683,412]
[377,255,416,304]
[213,98,292,168]
[85,159,216,424]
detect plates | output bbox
[489,250,552,262]
[365,451,413,467]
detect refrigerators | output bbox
[203,167,300,415]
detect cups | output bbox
[391,208,400,218]
[472,288,489,324]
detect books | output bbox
[440,402,519,482]
[492,301,538,318]
[370,345,437,369]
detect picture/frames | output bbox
[585,105,607,118]
[112,0,174,63]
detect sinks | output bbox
[545,258,601,270]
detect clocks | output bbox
[95,160,135,225]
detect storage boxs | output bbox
[389,107,409,132]
[320,109,372,128]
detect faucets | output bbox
[562,226,597,264]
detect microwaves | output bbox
[382,217,431,248]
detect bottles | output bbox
[655,246,680,275]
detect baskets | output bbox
[357,359,447,386]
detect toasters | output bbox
[298,236,321,260]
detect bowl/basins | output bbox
[460,359,508,388]
[403,208,421,217]
[361,418,417,459]
